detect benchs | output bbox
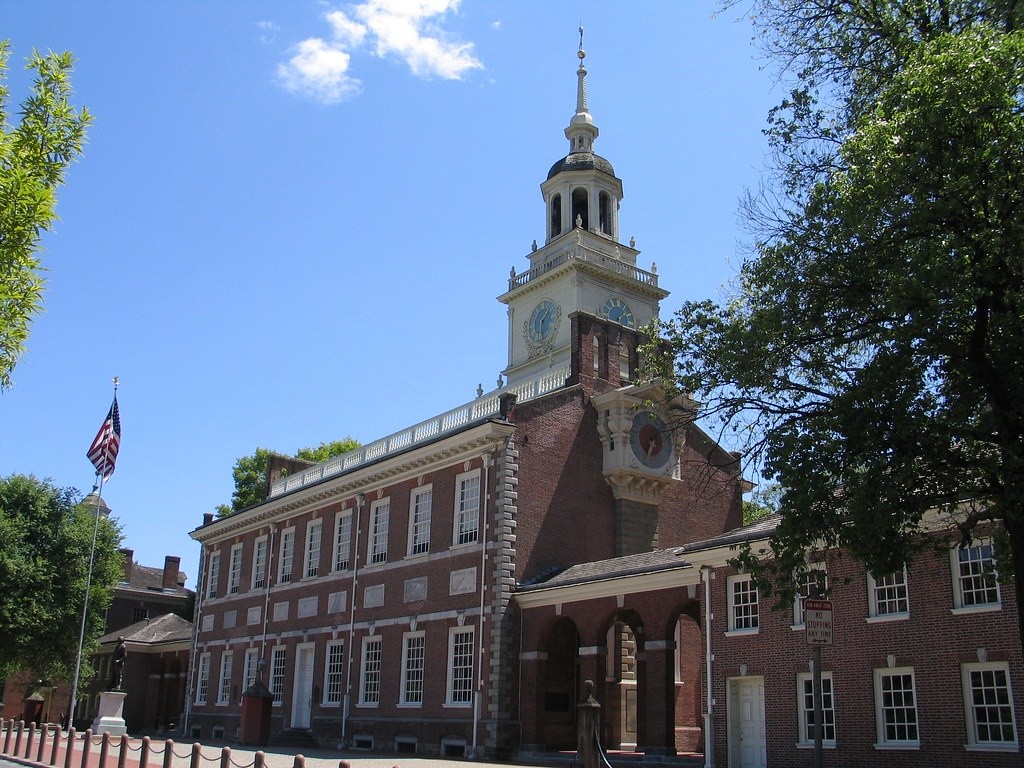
[39,722,58,731]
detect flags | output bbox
[87,397,121,484]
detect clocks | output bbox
[629,411,671,469]
[599,294,637,330]
[528,298,558,343]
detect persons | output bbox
[110,636,127,690]
